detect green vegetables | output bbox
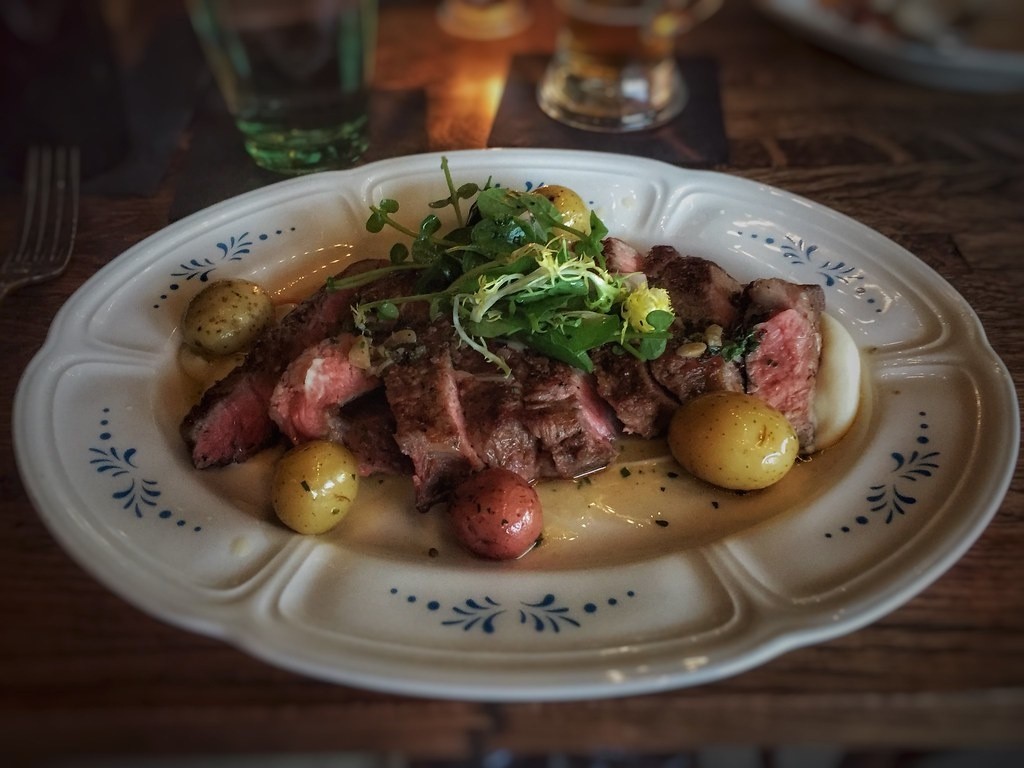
[327,154,673,371]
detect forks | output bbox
[0,143,88,305]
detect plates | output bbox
[763,0,1024,94]
[13,147,1020,702]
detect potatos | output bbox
[271,442,360,535]
[667,391,798,489]
[534,184,588,239]
[453,470,539,558]
[182,279,273,352]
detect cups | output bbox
[186,0,379,174]
[535,0,725,133]
